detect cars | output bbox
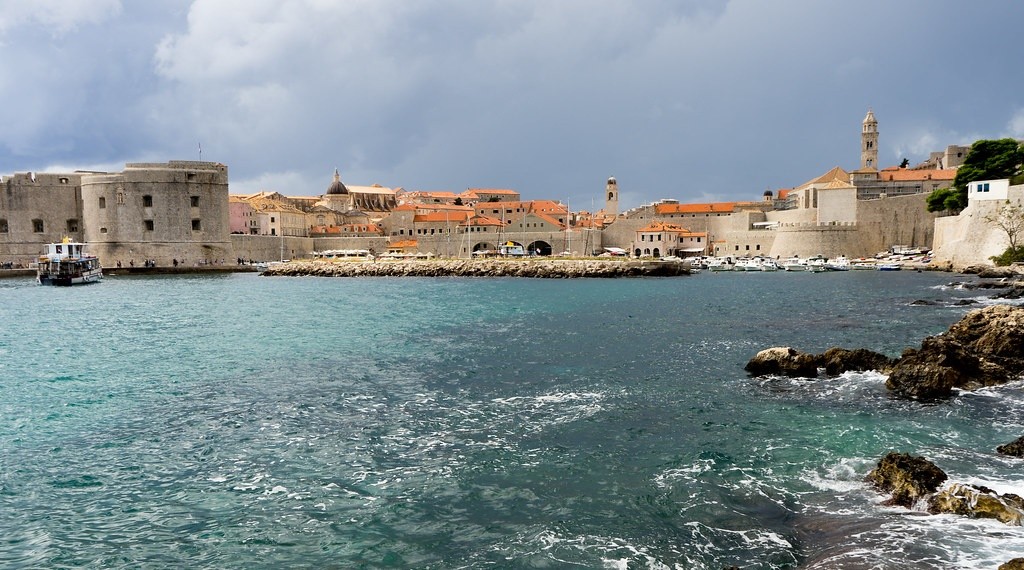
[21,260,37,268]
[1,260,20,269]
[664,256,681,261]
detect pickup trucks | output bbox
[611,251,625,256]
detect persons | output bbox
[145,259,151,268]
[237,258,246,265]
[205,258,224,266]
[117,261,121,268]
[130,259,133,267]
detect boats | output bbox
[743,260,762,271]
[36,236,103,286]
[760,261,778,271]
[824,257,852,271]
[734,258,749,271]
[878,264,900,270]
[686,256,734,274]
[783,259,806,271]
[809,260,826,272]
[853,263,877,270]
